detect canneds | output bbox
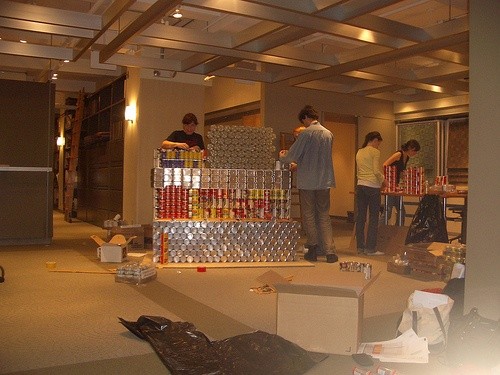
[352,366,372,375]
[377,365,396,375]
[153,124,302,263]
[116,262,152,279]
[445,244,466,264]
[384,165,456,194]
[340,261,372,279]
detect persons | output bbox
[162,113,204,152]
[278,105,338,263]
[383,139,420,226]
[356,131,391,256]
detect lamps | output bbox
[173,5,183,18]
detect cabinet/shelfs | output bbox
[0,73,127,247]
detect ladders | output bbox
[64,85,86,223]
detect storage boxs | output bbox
[258,269,382,358]
[90,213,153,263]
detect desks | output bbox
[352,190,468,246]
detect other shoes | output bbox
[326,254,339,263]
[304,250,318,261]
[357,248,385,256]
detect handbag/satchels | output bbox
[393,289,455,353]
[448,308,499,370]
